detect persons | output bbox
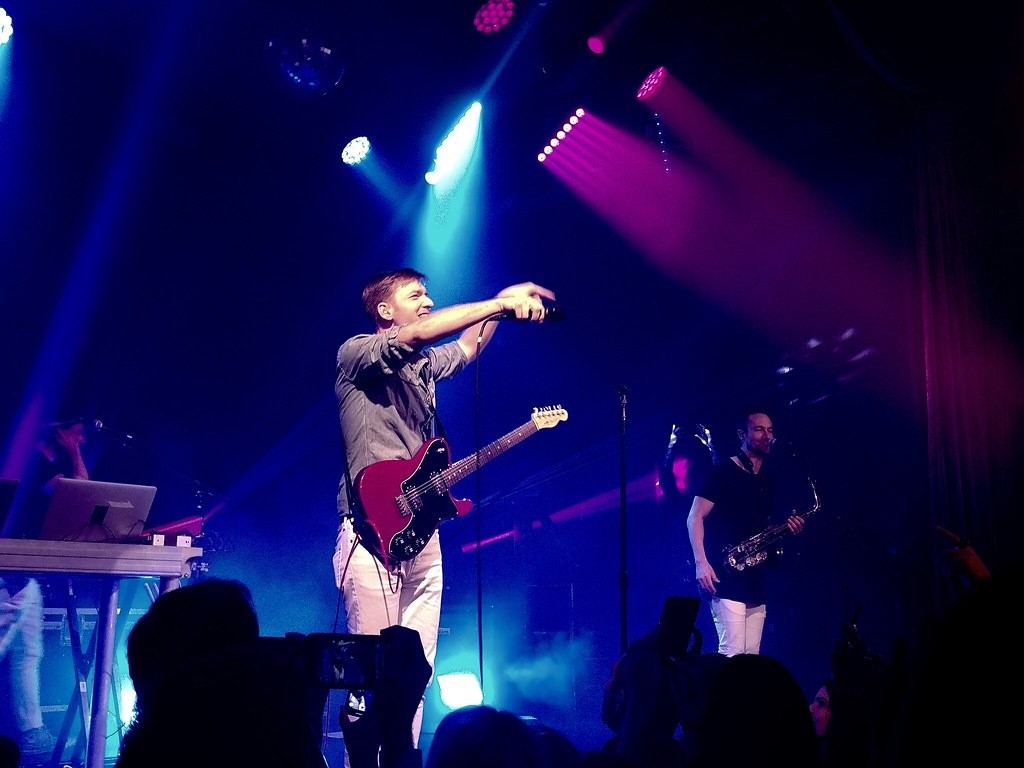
[687,411,805,657]
[424,524,1024,768]
[116,576,432,768]
[0,399,90,757]
[332,267,557,767]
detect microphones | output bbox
[92,420,133,445]
[769,437,792,446]
[490,307,562,322]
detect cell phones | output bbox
[306,632,381,691]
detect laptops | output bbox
[38,478,157,543]
[0,478,20,530]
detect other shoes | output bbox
[21,727,77,755]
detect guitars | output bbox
[351,403,571,566]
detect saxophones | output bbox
[722,479,828,576]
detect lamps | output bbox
[332,126,371,166]
[466,0,515,33]
